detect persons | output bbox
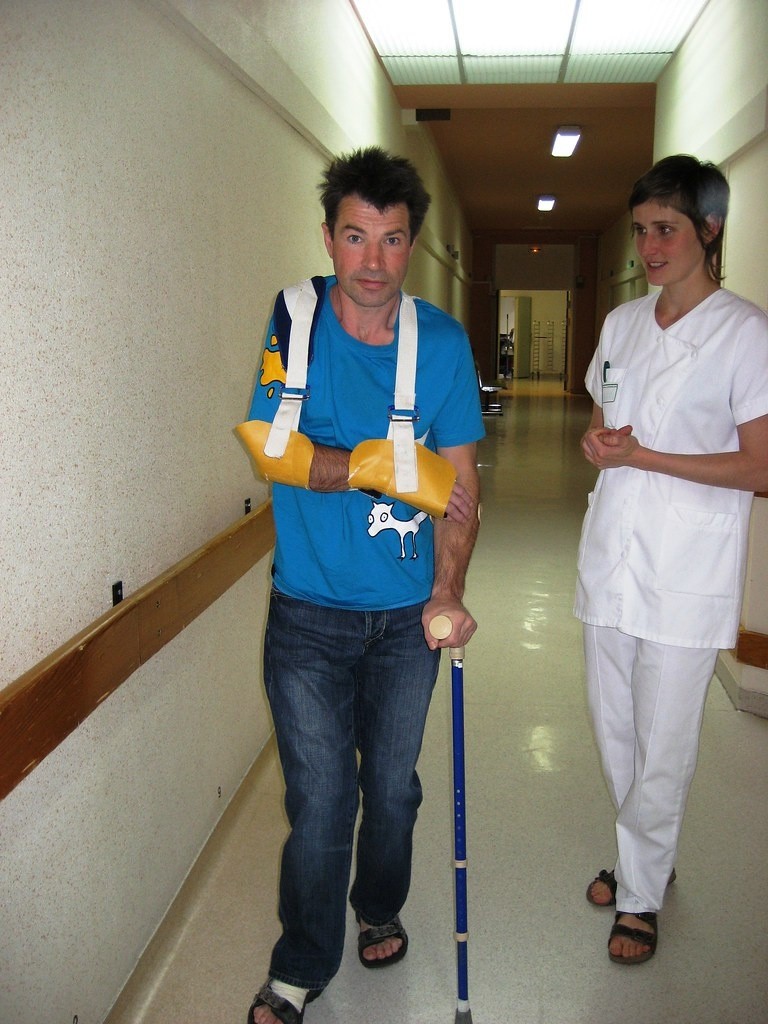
[239,148,486,1023]
[580,154,768,965]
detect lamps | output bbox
[538,194,557,211]
[552,126,582,158]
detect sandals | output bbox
[248,975,324,1024]
[354,910,409,969]
[586,861,677,906]
[607,908,658,965]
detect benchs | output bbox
[475,359,506,417]
